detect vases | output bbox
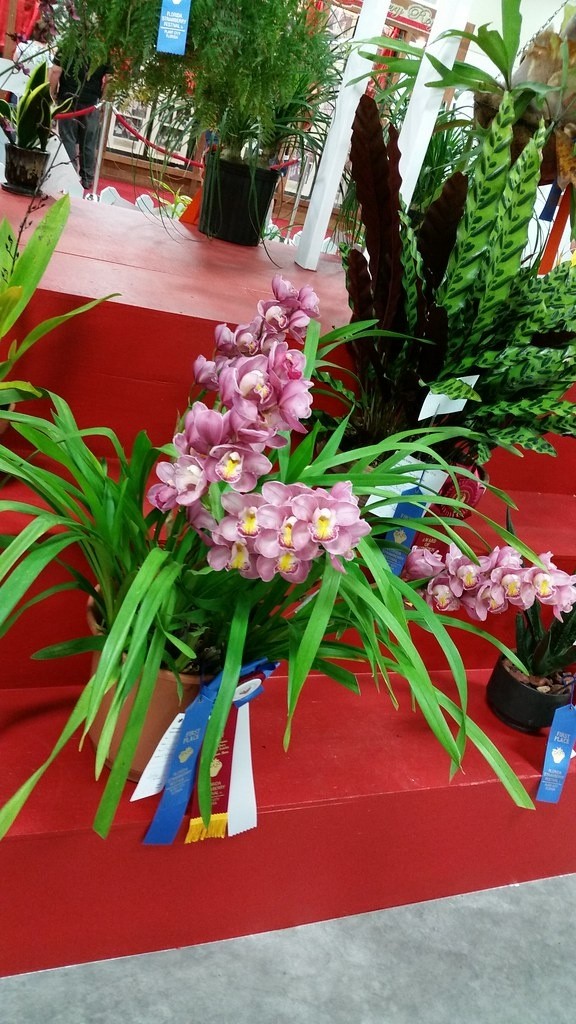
[85,587,220,785]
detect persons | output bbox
[12,15,115,189]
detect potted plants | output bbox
[0,59,74,197]
[486,501,576,733]
[38,0,576,592]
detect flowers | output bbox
[1,278,576,842]
